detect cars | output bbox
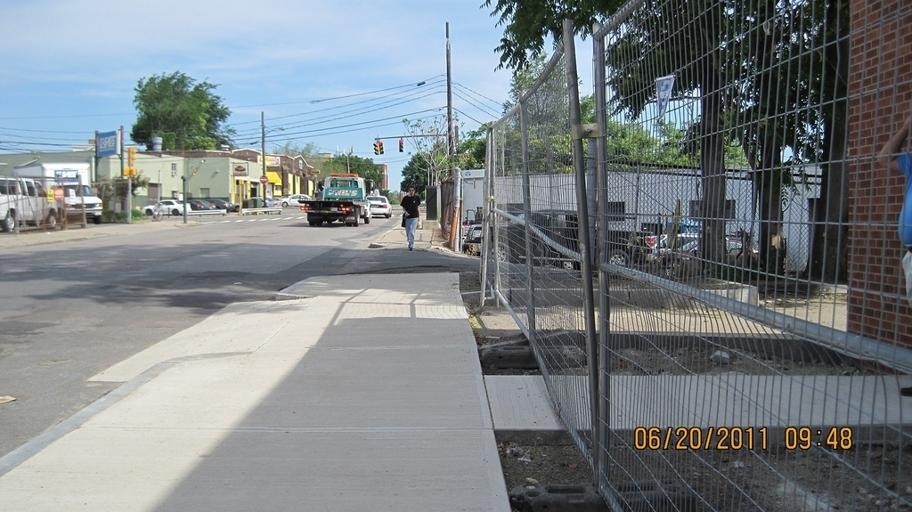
[143,198,236,217]
[366,195,392,219]
[275,194,310,209]
[651,233,757,277]
[235,197,281,210]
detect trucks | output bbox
[299,174,372,227]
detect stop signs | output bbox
[261,176,267,185]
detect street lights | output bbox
[262,127,285,172]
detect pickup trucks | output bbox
[488,208,653,270]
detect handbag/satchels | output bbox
[401,213,407,227]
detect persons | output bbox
[400,186,421,251]
[877,113,912,397]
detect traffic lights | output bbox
[373,142,379,156]
[129,149,138,170]
[399,140,403,152]
[379,142,384,154]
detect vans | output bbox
[1,177,103,232]
[468,225,499,244]
